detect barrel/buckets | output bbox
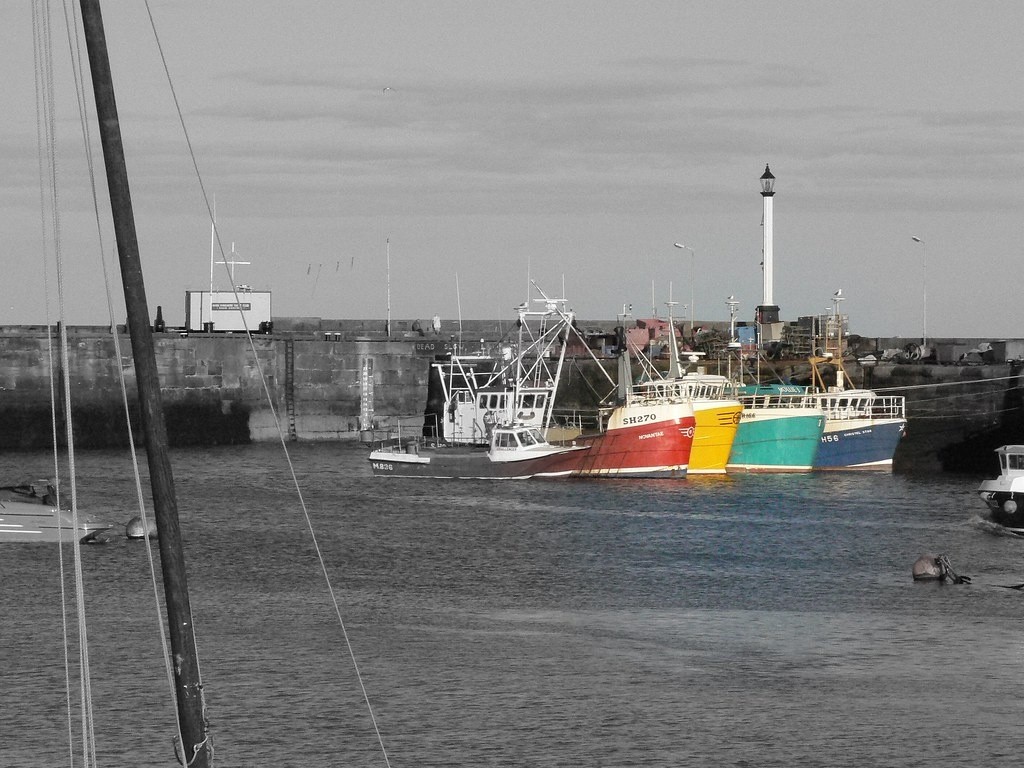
[204,322,214,333]
[262,322,273,335]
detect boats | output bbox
[1,478,114,545]
[977,444,1024,529]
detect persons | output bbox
[412,319,425,337]
[432,313,441,335]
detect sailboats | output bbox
[368,253,908,481]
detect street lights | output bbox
[911,234,927,346]
[755,162,786,340]
[674,242,694,342]
